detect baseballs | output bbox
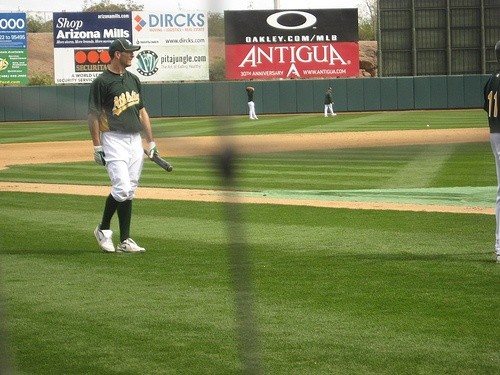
[426,125,429,127]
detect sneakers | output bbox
[94,224,115,252]
[117,238,145,252]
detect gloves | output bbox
[147,142,160,164]
[93,145,106,166]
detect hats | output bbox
[109,39,141,56]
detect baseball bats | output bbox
[143,149,173,172]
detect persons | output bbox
[88,38,159,253]
[246,87,258,120]
[324,87,336,117]
[483,40,500,263]
